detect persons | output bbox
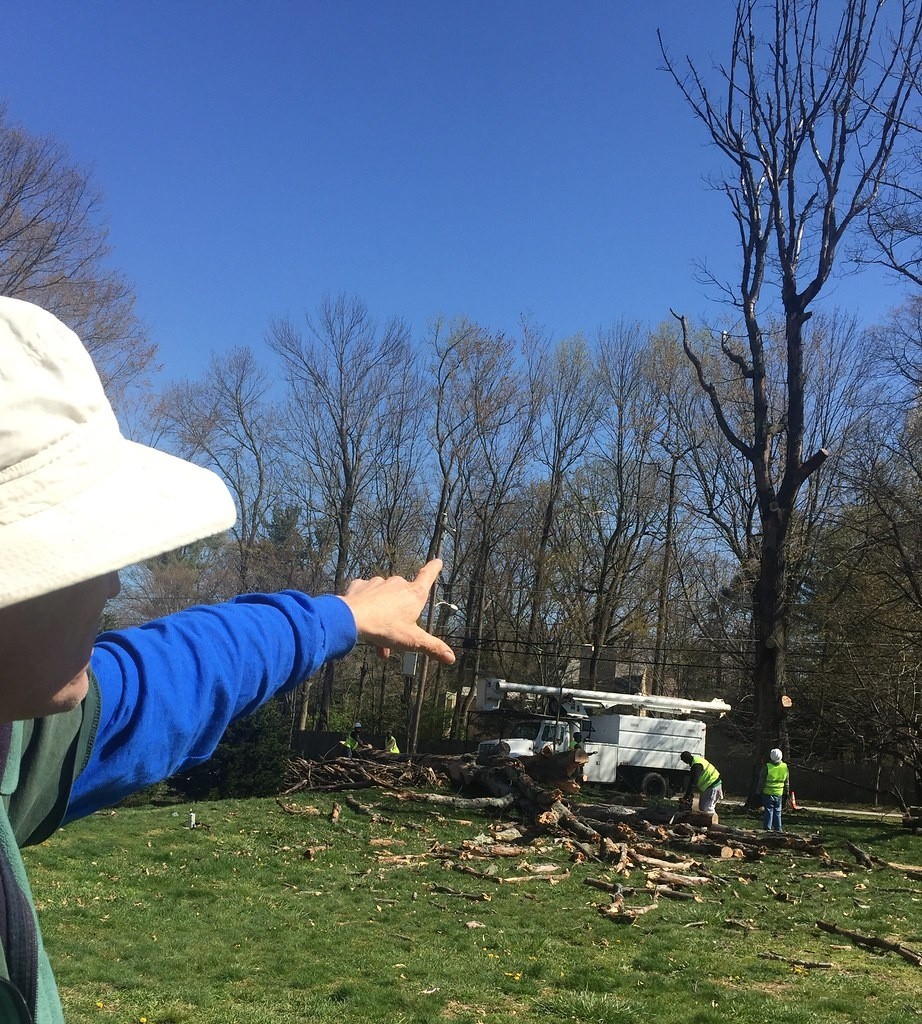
[757,748,790,832]
[678,750,723,812]
[569,732,599,779]
[380,729,400,754]
[343,722,364,759]
[0,295,460,1023]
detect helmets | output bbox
[770,748,783,763]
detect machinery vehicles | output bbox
[473,676,732,800]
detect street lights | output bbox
[410,602,458,755]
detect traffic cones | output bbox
[790,791,798,811]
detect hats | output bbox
[1,294,237,619]
